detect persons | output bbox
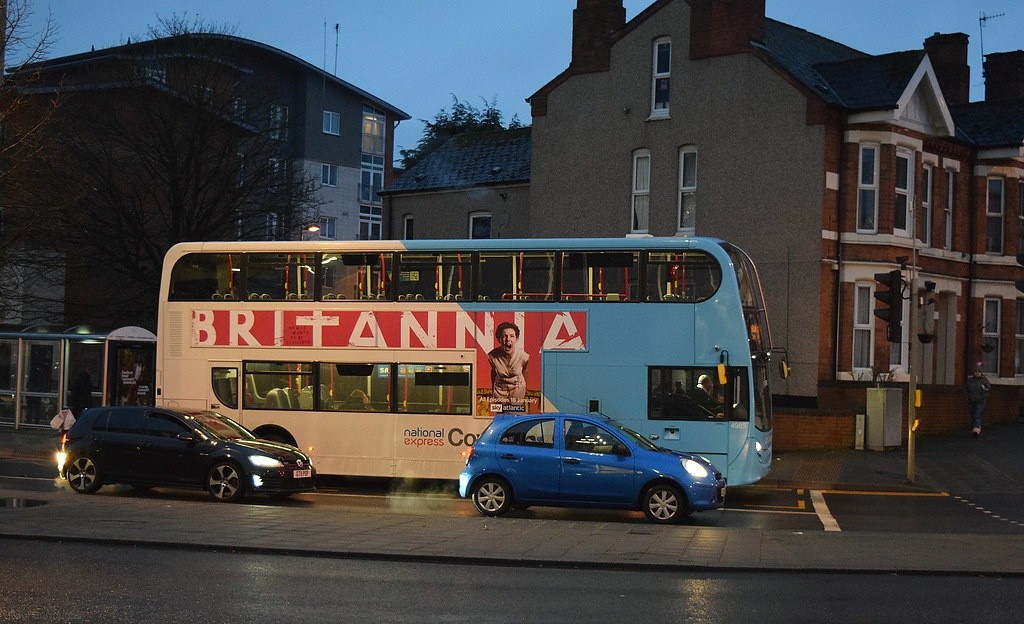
[70,366,92,420]
[25,368,47,425]
[578,426,606,445]
[695,375,724,418]
[487,322,530,414]
[965,362,990,435]
[339,389,370,410]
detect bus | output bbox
[155,236,792,487]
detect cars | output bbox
[57,405,318,504]
[459,412,726,524]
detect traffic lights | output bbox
[873,270,901,344]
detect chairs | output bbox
[300,294,309,299]
[337,293,346,300]
[287,293,299,299]
[415,294,424,301]
[326,293,337,299]
[320,384,334,410]
[264,388,290,409]
[405,294,415,301]
[368,293,378,300]
[447,293,455,301]
[283,387,300,408]
[455,294,463,301]
[211,293,222,299]
[377,294,386,300]
[248,293,261,299]
[223,294,234,300]
[261,294,272,300]
[398,295,407,301]
